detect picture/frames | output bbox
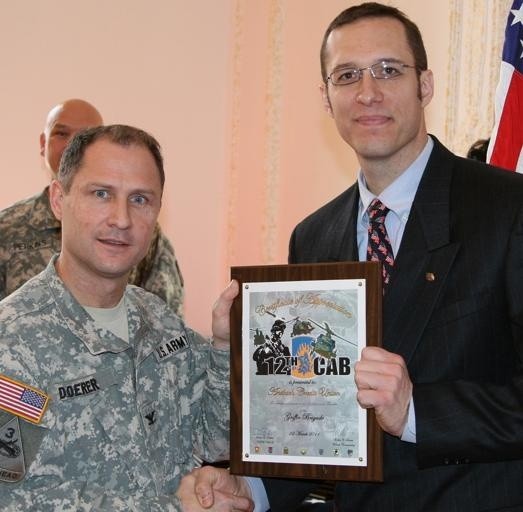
[229,259,387,484]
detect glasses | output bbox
[325,61,416,86]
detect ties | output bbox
[366,198,395,297]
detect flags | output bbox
[486,0,523,173]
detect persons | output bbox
[0,124,256,512]
[0,98,184,319]
[192,0,522,511]
